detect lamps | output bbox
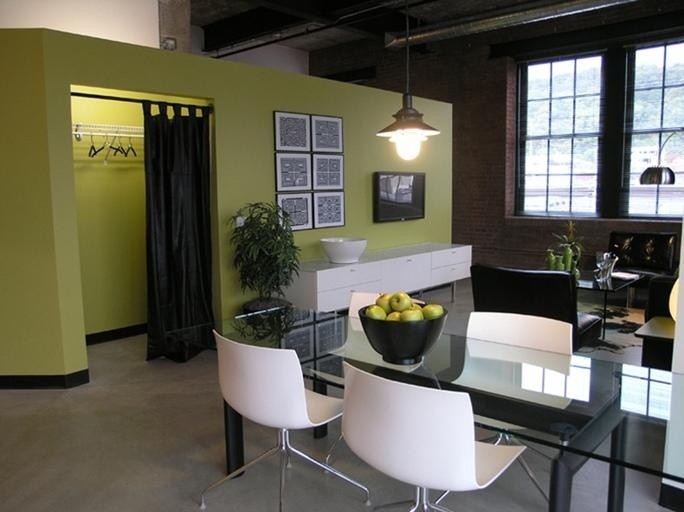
[640,130,684,184]
[376,13,440,161]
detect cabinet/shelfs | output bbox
[279,241,474,314]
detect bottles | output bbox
[544,245,579,275]
[593,244,619,280]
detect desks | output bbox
[222,304,683,511]
[576,271,646,340]
[635,317,675,371]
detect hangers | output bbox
[88,123,138,164]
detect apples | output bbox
[411,304,424,320]
[390,292,412,311]
[386,311,401,321]
[366,305,386,320]
[401,311,418,321]
[423,304,444,319]
[376,292,393,313]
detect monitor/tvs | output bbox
[372,170,427,223]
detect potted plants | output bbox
[225,202,302,325]
[550,216,587,286]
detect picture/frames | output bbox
[313,192,345,229]
[311,114,344,154]
[275,193,313,231]
[273,110,312,153]
[312,153,343,191]
[279,309,346,374]
[274,152,312,192]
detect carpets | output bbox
[577,304,645,366]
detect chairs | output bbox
[341,360,528,512]
[608,231,678,289]
[200,329,372,512]
[470,264,602,353]
[323,292,398,475]
[435,311,578,503]
